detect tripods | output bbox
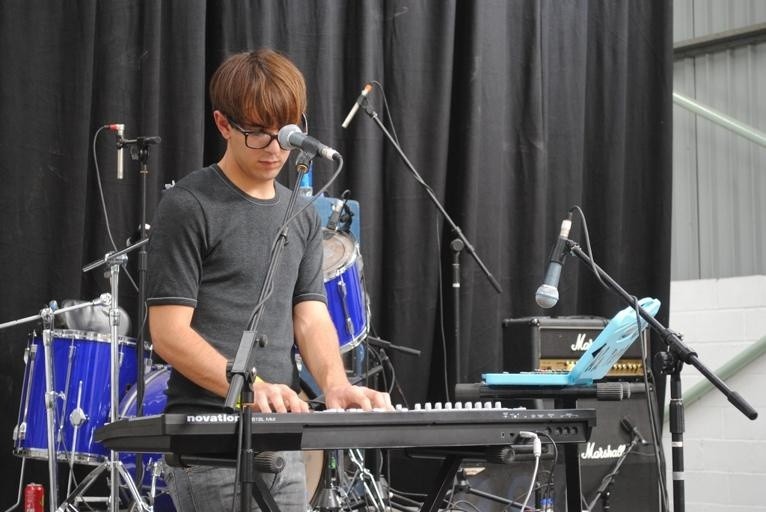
[52,314,150,512]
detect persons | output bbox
[142,49,394,512]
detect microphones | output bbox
[278,124,338,163]
[536,211,574,309]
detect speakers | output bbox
[503,317,663,510]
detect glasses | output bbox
[227,112,308,150]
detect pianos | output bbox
[91,401,597,456]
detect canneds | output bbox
[24,481,45,512]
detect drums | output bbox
[13,326,153,467]
[293,227,371,361]
[109,363,176,512]
